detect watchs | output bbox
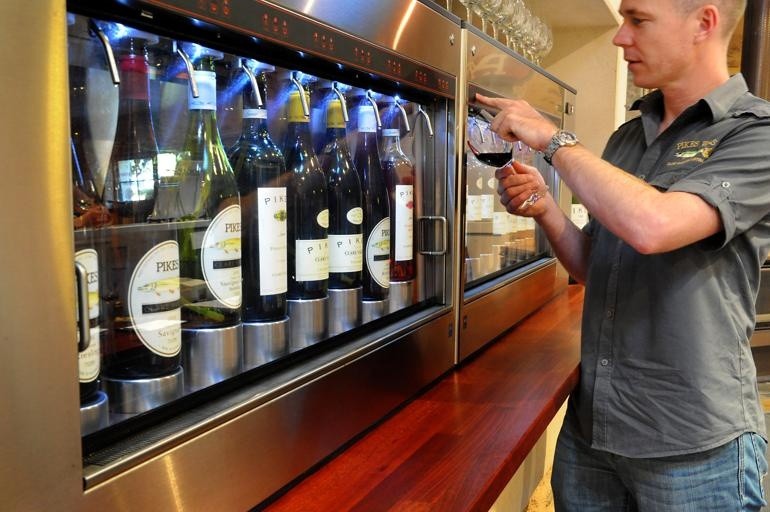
[543,129,579,164]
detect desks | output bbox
[256,283,586,512]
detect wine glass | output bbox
[445,0,554,68]
[467,113,550,213]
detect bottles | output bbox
[377,100,421,282]
[69,36,185,404]
[320,90,366,290]
[280,78,332,301]
[353,91,394,301]
[178,46,244,333]
[464,148,537,260]
[226,68,290,324]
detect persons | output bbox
[474,0,770,511]
